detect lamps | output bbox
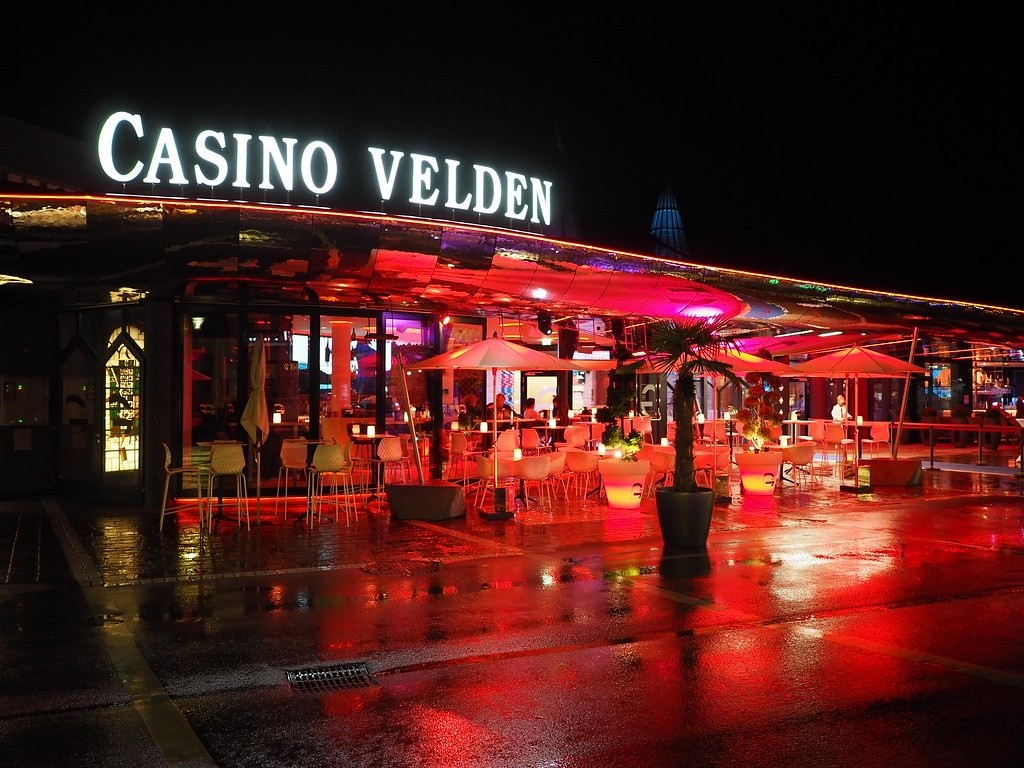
[698,413,704,424]
[723,411,730,419]
[549,418,556,428]
[592,408,597,414]
[568,409,575,418]
[660,438,668,446]
[856,416,863,426]
[790,413,797,421]
[403,412,409,422]
[779,436,787,446]
[513,449,522,461]
[598,444,606,455]
[451,422,458,431]
[367,425,375,438]
[628,410,635,420]
[272,413,282,423]
[440,312,451,325]
[352,424,360,434]
[480,422,488,432]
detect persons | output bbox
[797,394,804,409]
[552,398,557,419]
[524,398,550,443]
[458,394,482,430]
[831,395,852,449]
[486,394,516,428]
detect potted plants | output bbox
[735,349,785,496]
[638,312,751,547]
[597,423,651,510]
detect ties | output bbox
[841,409,843,418]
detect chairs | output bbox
[207,439,251,533]
[861,422,892,458]
[159,442,205,530]
[321,404,864,510]
[305,445,351,529]
[275,437,311,521]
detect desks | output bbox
[782,420,811,444]
[578,414,596,422]
[533,426,566,453]
[268,422,309,439]
[350,434,396,491]
[486,419,535,437]
[554,417,580,424]
[461,431,502,457]
[623,416,641,432]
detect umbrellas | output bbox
[406,332,587,488]
[625,344,806,477]
[773,346,931,430]
[240,330,270,524]
